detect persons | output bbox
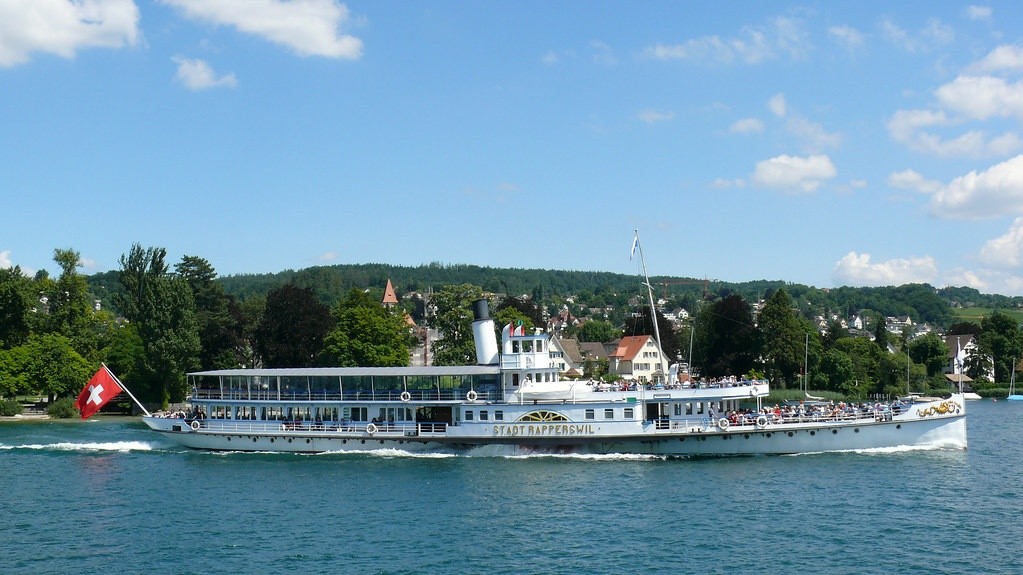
[164,407,205,419]
[587,375,762,393]
[709,395,912,429]
[279,416,396,431]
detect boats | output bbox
[1007,356,1023,400]
[964,393,983,399]
[142,228,968,457]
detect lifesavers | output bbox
[366,423,376,435]
[718,418,729,430]
[400,391,410,402]
[750,385,758,397]
[466,391,478,402]
[757,416,767,428]
[191,421,200,431]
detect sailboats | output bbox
[896,349,919,402]
[782,333,831,407]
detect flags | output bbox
[629,236,638,262]
[72,367,124,421]
